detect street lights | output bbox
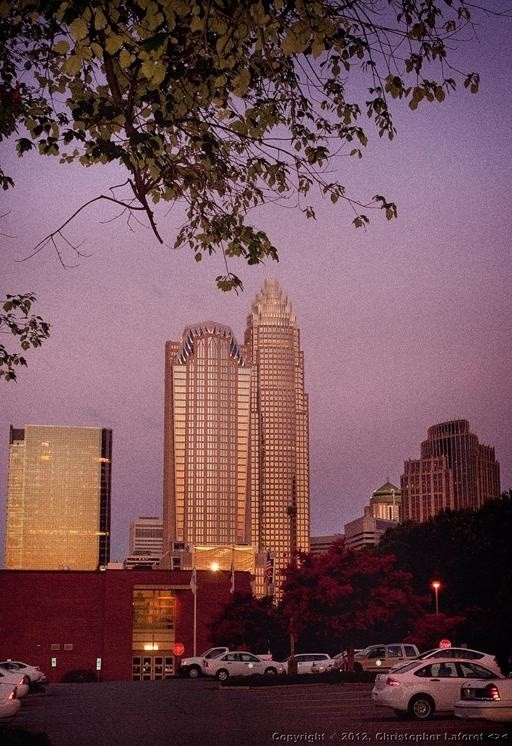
[433,579,441,615]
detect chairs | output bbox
[438,666,451,677]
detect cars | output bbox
[179,642,512,722]
[0,658,47,719]
